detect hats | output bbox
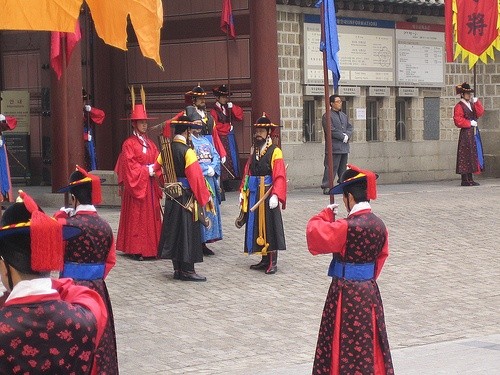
[214,83,232,96]
[0,190,81,273]
[170,110,202,127]
[82,88,87,100]
[56,165,106,204]
[254,112,284,128]
[185,81,207,97]
[330,164,379,200]
[456,82,474,95]
[120,105,159,120]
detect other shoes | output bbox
[202,243,214,254]
[323,188,330,194]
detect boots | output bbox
[250,251,277,274]
[461,175,480,186]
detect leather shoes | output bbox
[174,270,207,281]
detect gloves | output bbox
[0,114,5,121]
[149,164,155,176]
[239,193,244,202]
[343,133,348,143]
[269,195,279,210]
[471,119,478,126]
[208,166,215,177]
[327,203,338,215]
[85,105,91,111]
[0,137,3,146]
[88,130,92,142]
[472,97,477,102]
[221,156,226,163]
[60,206,74,214]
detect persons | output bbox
[0,113,17,202]
[306,164,394,375]
[116,83,243,282]
[240,112,287,274]
[0,190,108,375]
[454,82,486,186]
[55,165,119,375]
[82,88,105,172]
[321,95,353,195]
